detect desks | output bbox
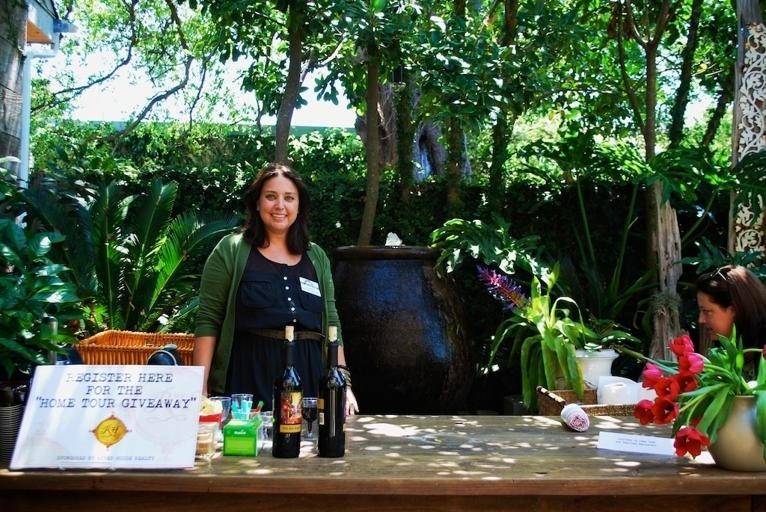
[1,415,766,512]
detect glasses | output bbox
[710,267,728,281]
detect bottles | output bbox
[318,325,345,457]
[272,321,303,458]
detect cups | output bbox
[194,430,214,466]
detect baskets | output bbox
[76,329,195,364]
[536,385,635,416]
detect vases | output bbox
[706,395,766,472]
[544,346,621,390]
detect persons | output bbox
[191,161,361,418]
[695,263,766,382]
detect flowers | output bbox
[632,321,766,459]
[475,259,644,411]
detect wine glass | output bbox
[209,393,273,442]
[301,397,319,438]
